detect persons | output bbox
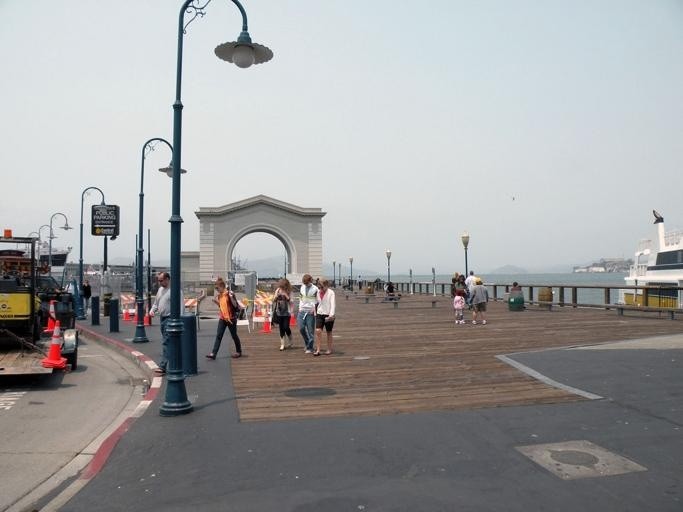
[451,272,460,282]
[464,271,478,310]
[272,279,293,351]
[468,280,489,325]
[387,282,395,300]
[204,277,241,359]
[82,279,91,315]
[295,273,319,353]
[312,277,336,356]
[148,272,184,373]
[510,281,526,309]
[357,276,362,289]
[454,275,469,311]
[453,289,466,324]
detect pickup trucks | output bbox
[24,275,78,332]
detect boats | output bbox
[617,209,683,308]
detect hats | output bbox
[475,277,481,282]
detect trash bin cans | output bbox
[104,292,112,316]
[538,287,553,308]
[508,290,524,311]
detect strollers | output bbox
[463,288,476,311]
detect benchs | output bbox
[335,284,387,304]
[523,297,682,318]
[380,298,440,309]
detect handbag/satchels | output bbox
[272,309,281,325]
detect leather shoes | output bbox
[153,367,166,373]
[287,343,294,347]
[279,345,286,350]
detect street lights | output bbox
[131,136,187,345]
[384,249,392,283]
[461,230,469,278]
[349,256,353,291]
[26,213,72,276]
[77,186,104,320]
[159,0,273,416]
[338,259,342,286]
[332,259,337,287]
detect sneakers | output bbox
[471,320,477,325]
[455,320,467,324]
[203,352,217,360]
[482,320,486,325]
[230,351,241,358]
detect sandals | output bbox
[303,347,335,356]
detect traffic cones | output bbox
[43,299,57,333]
[42,320,68,369]
[289,302,297,326]
[143,303,149,325]
[121,303,131,321]
[260,312,271,333]
[131,303,138,323]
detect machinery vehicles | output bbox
[0,225,43,346]
